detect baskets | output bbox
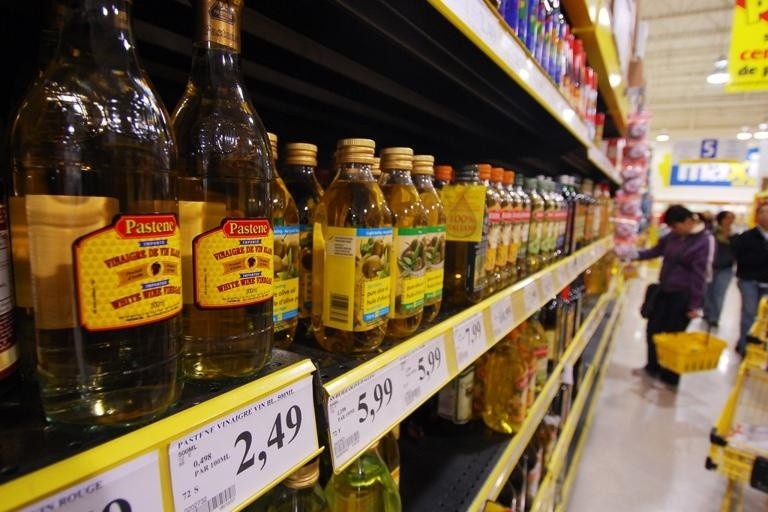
[652,313,727,374]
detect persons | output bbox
[703,210,739,325]
[634,204,718,391]
[734,202,768,358]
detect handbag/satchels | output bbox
[640,283,661,318]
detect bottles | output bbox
[266,131,299,350]
[377,430,403,485]
[10,0,185,430]
[324,442,402,512]
[377,146,427,336]
[170,0,276,376]
[370,156,381,177]
[437,367,474,424]
[313,138,393,352]
[411,153,447,322]
[444,163,579,303]
[281,142,323,339]
[576,180,616,248]
[267,457,330,512]
[432,166,451,197]
[473,309,548,434]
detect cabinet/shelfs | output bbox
[0,1,630,512]
